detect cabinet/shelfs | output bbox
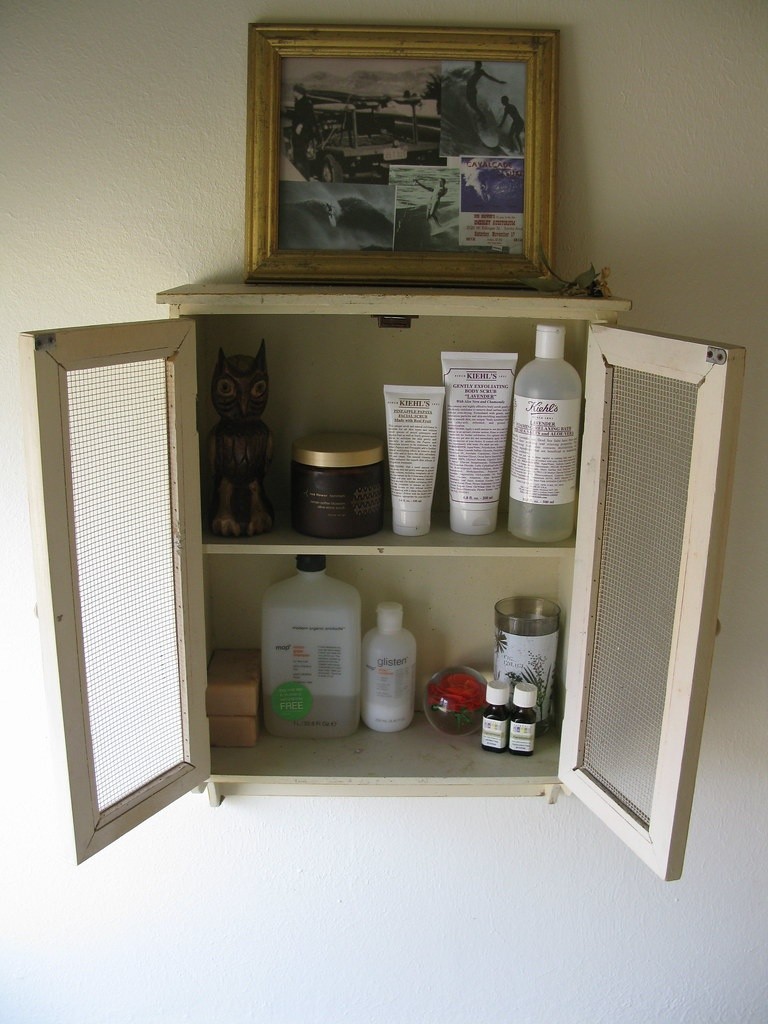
[16,277,748,881]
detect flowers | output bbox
[428,673,487,732]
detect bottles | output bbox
[481,680,510,753]
[509,682,538,756]
[261,555,361,738]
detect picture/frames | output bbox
[243,23,561,290]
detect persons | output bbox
[498,96,526,155]
[291,83,313,178]
[460,60,507,115]
[322,203,335,217]
[412,176,447,228]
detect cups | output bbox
[495,596,561,737]
